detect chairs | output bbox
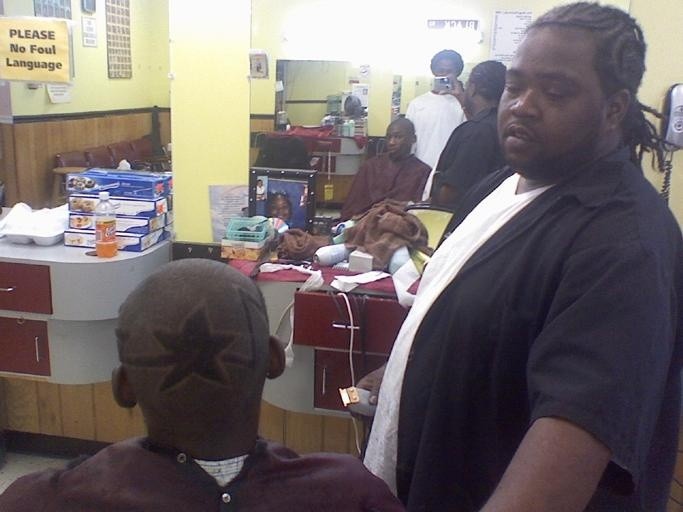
[50,137,171,206]
[405,205,456,251]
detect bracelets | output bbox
[460,104,473,113]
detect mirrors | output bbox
[0,0,169,221]
[273,59,350,129]
[249,2,630,275]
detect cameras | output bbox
[432,77,451,92]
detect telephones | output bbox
[663,82,683,153]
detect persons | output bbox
[264,191,292,227]
[340,118,432,220]
[362,0,681,511]
[415,61,510,203]
[255,179,265,201]
[0,258,402,511]
[404,49,474,202]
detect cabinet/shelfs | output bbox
[0,236,171,386]
[228,258,414,419]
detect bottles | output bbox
[323,178,334,202]
[93,191,116,260]
[341,119,355,139]
